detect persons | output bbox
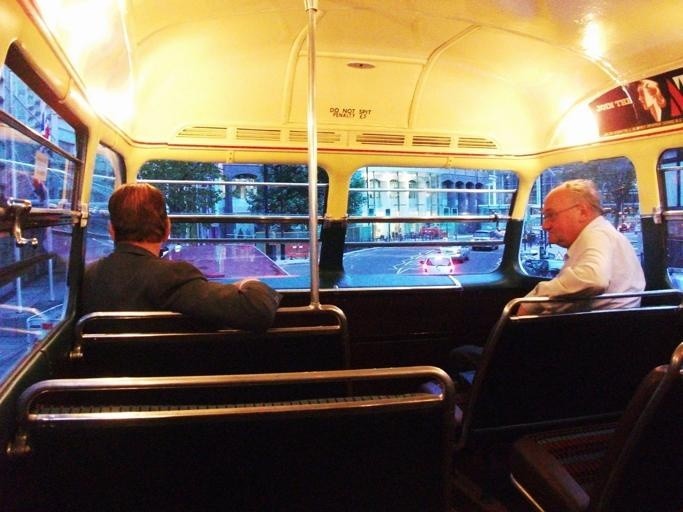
[515,177,647,316]
[636,78,669,126]
[76,182,283,334]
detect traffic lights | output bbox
[451,208,458,216]
[443,207,449,216]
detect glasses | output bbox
[542,203,590,221]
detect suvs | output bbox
[470,229,499,250]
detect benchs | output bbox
[0,283,683,512]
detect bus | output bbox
[477,203,543,238]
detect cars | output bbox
[438,245,470,262]
[619,224,630,232]
[420,253,456,275]
